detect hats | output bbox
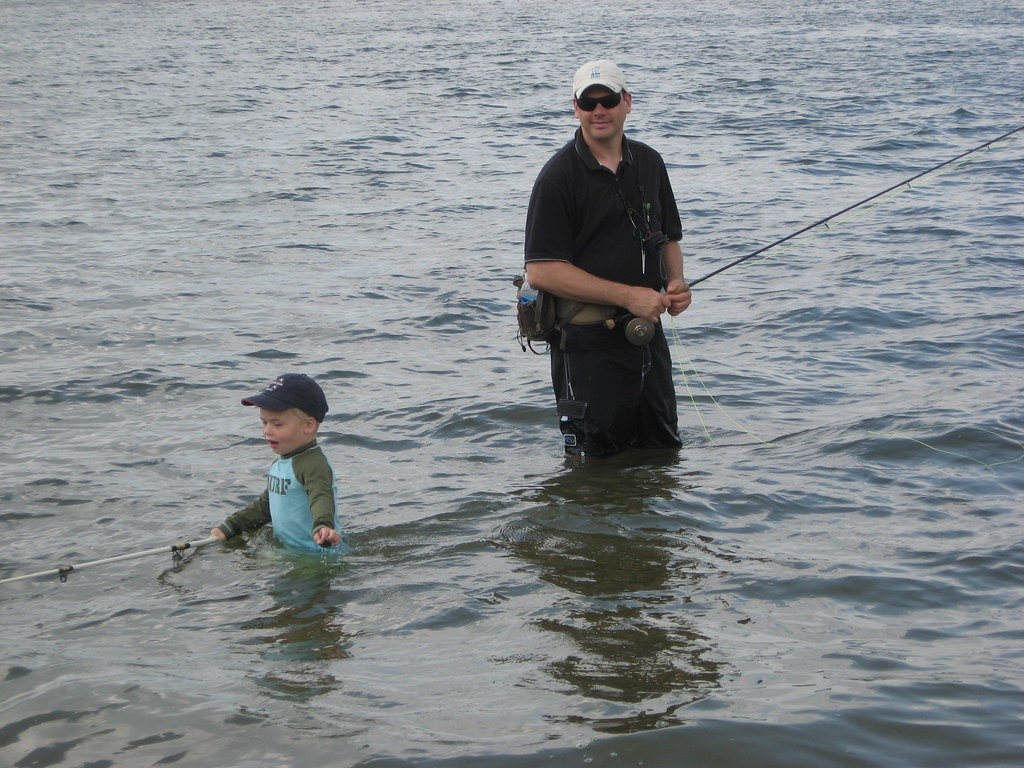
[572,59,630,100]
[241,373,328,423]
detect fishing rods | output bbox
[602,126,1024,346]
[0,536,221,585]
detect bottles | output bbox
[520,273,538,305]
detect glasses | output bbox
[576,92,623,112]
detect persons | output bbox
[211,372,350,554]
[524,60,692,454]
